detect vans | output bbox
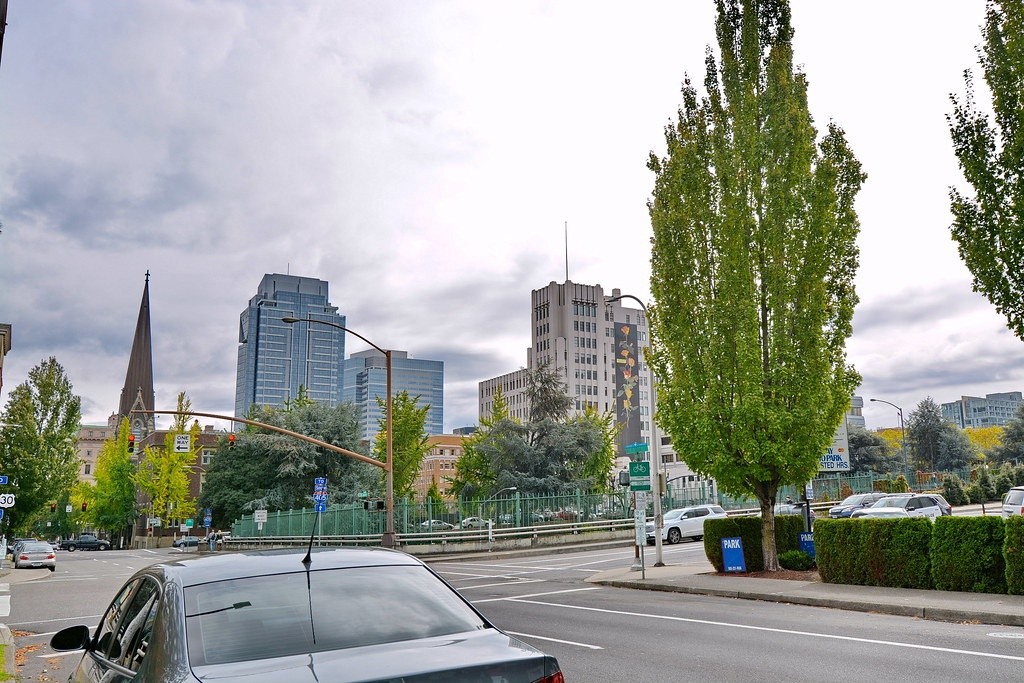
[204,531,232,544]
[1001,485,1024,521]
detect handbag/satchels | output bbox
[217,539,222,543]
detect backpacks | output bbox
[211,533,215,540]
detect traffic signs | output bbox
[174,435,190,453]
[66,505,72,512]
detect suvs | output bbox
[828,492,952,518]
[645,504,729,545]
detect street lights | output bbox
[870,398,908,481]
[479,487,517,551]
[280,316,395,548]
[606,294,663,566]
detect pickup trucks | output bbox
[59,535,111,552]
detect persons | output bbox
[208,530,216,551]
[786,496,792,504]
[215,530,222,551]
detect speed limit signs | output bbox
[0,494,16,507]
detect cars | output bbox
[7,538,59,572]
[419,519,454,531]
[752,505,816,526]
[50,545,565,683]
[463,506,615,528]
[172,536,201,547]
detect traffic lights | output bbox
[377,501,385,510]
[148,527,152,531]
[82,503,87,512]
[51,504,56,513]
[228,433,236,452]
[363,501,373,510]
[128,433,135,453]
